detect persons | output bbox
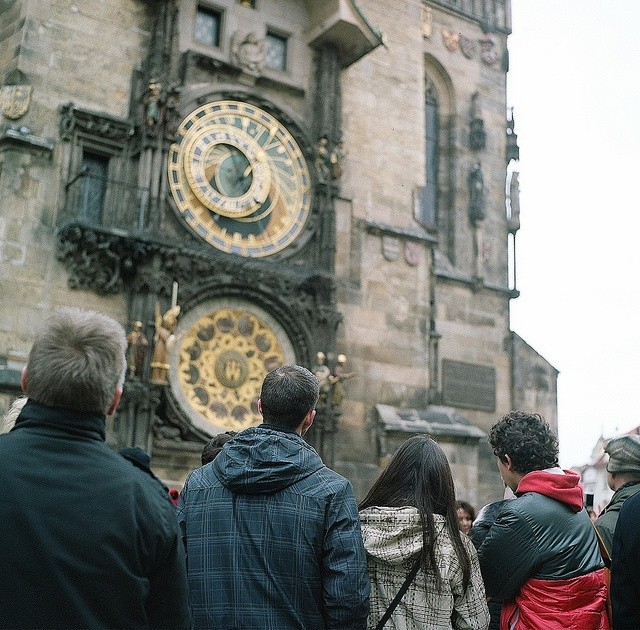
[611,489,638,630]
[586,508,598,523]
[3,305,196,628]
[354,437,492,630]
[454,498,477,534]
[589,434,639,630]
[179,363,369,630]
[471,410,610,630]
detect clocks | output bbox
[168,100,314,258]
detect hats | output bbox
[603,435,640,473]
